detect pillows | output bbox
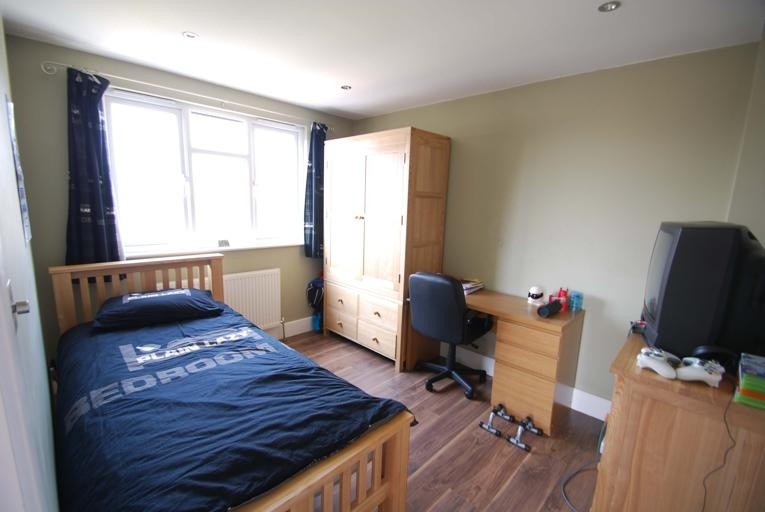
[93,287,225,331]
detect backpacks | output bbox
[305,277,324,316]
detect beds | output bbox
[48,254,411,512]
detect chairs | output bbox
[408,271,495,400]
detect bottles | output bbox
[528,287,581,317]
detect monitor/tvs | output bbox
[641,221,765,375]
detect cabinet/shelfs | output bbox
[324,127,451,371]
[587,331,765,512]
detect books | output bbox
[463,285,485,296]
[461,280,484,290]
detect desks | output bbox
[407,274,584,439]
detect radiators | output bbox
[157,268,283,334]
